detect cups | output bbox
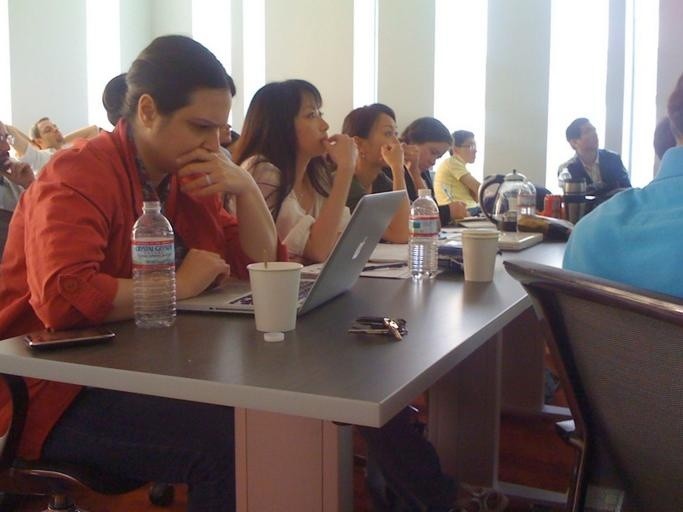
[460,229,500,282]
[245,261,303,332]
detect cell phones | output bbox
[23,323,115,350]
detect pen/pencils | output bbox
[444,187,455,201]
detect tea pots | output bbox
[478,169,537,231]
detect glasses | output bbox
[0,132,16,146]
[455,141,476,149]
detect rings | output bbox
[206,174,213,187]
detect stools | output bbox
[0,460,93,512]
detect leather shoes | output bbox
[451,479,510,512]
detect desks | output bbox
[0,205,683,511]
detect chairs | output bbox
[502,256,683,511]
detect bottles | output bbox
[558,167,572,196]
[514,176,535,233]
[406,187,442,280]
[131,201,178,328]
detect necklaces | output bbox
[297,191,304,203]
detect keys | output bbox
[347,314,407,341]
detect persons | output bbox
[222,117,240,150]
[6,116,99,181]
[0,125,36,250]
[562,75,683,298]
[382,117,469,226]
[432,129,482,218]
[228,79,449,511]
[653,116,676,161]
[1,36,288,510]
[332,103,430,245]
[558,117,632,213]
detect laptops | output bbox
[175,189,407,318]
